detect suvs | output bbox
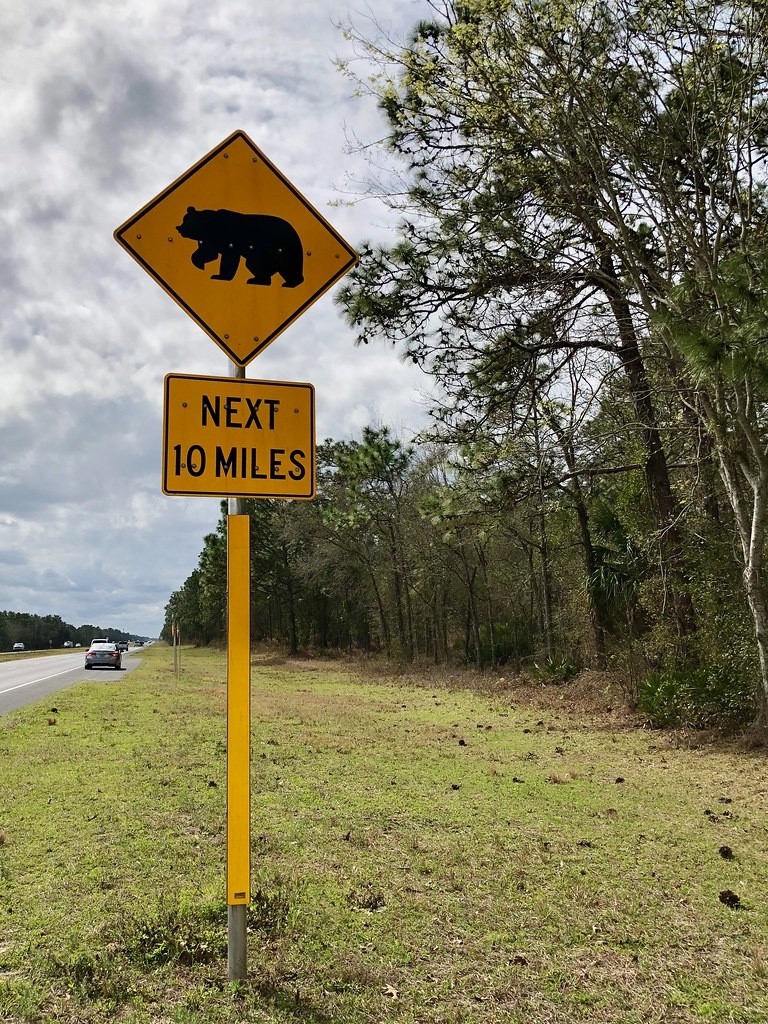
[90,638,109,647]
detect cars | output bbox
[12,643,25,651]
[117,641,129,651]
[84,643,123,670]
[134,641,141,647]
